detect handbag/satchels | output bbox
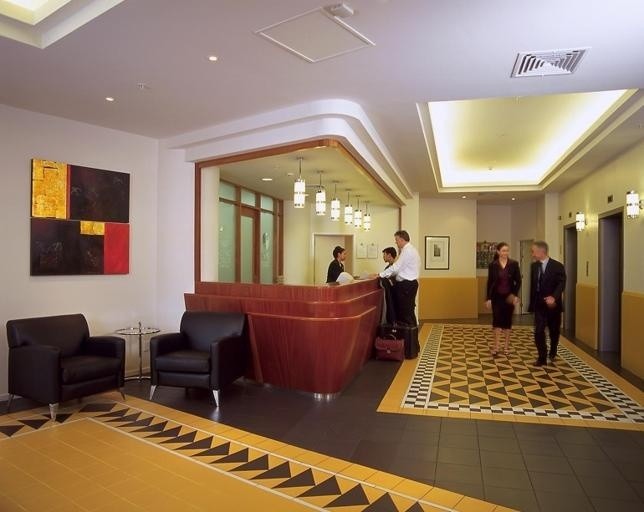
[374,335,404,361]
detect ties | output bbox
[539,263,544,286]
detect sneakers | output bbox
[532,354,556,366]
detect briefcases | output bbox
[382,320,418,358]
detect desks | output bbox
[116,321,159,380]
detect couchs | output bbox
[5,313,126,421]
[148,309,251,406]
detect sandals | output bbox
[492,347,509,356]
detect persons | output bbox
[382,247,397,324]
[527,241,566,366]
[327,246,347,282]
[367,231,420,352]
[486,242,521,355]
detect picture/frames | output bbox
[425,236,449,270]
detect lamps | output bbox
[626,190,643,221]
[293,156,371,232]
[575,211,587,232]
